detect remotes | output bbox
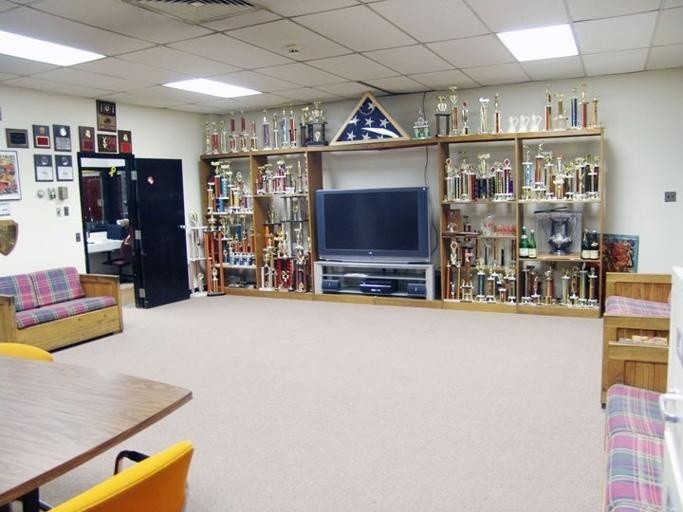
[344,272,367,278]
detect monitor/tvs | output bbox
[315,187,430,264]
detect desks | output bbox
[0,356,192,512]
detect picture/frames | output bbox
[0,150,22,201]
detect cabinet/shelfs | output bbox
[439,128,600,320]
[201,127,308,299]
[309,127,440,305]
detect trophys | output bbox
[446,212,600,310]
[592,98,600,129]
[434,94,460,138]
[544,92,552,131]
[412,109,432,140]
[522,144,598,201]
[204,101,326,292]
[461,102,469,135]
[493,96,502,134]
[570,88,578,129]
[444,153,513,202]
[479,98,490,135]
[554,95,568,131]
[580,92,590,129]
[508,115,542,133]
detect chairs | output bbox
[103,240,135,283]
[16,441,194,512]
[0,343,53,361]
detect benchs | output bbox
[600,272,671,407]
[0,266,124,351]
[605,339,672,512]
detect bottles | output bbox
[589,230,600,259]
[581,228,590,260]
[528,229,536,259]
[518,226,529,258]
[483,213,514,236]
